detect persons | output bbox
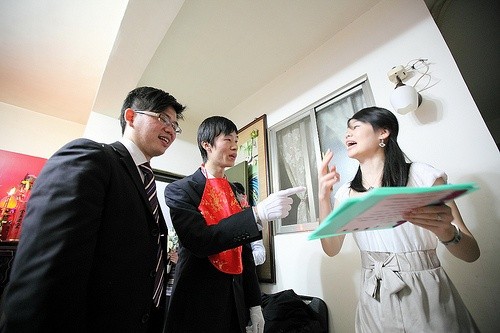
[0,87,186,333]
[318,106,480,333]
[164,116,307,333]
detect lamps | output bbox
[391,75,422,115]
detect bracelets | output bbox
[438,223,462,247]
